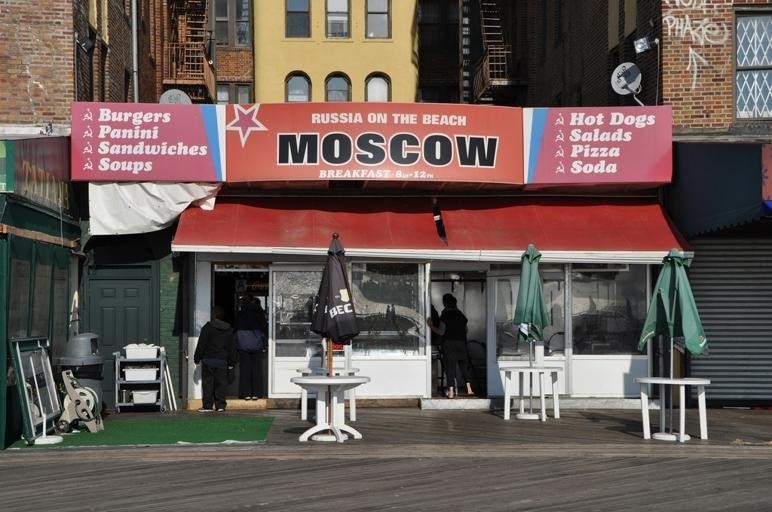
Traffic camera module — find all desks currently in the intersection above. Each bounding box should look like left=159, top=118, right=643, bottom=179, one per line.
left=291, top=376, right=371, bottom=443
left=500, top=366, right=564, bottom=420
left=634, top=376, right=710, bottom=443
left=296, top=367, right=360, bottom=422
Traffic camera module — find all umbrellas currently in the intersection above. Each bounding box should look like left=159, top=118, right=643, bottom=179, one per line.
left=638, top=248, right=709, bottom=435
left=512, top=244, right=551, bottom=416
left=310, top=233, right=362, bottom=435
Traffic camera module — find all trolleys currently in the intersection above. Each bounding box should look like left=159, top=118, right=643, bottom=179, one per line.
left=111, top=348, right=168, bottom=413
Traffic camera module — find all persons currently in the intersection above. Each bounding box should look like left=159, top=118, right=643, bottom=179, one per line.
left=427, top=293, right=475, bottom=399
left=238, top=296, right=267, bottom=400
left=193, top=304, right=239, bottom=413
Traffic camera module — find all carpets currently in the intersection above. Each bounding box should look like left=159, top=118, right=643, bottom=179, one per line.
left=8, top=416, right=275, bottom=448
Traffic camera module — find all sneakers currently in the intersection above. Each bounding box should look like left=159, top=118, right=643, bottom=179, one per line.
left=198, top=408, right=214, bottom=413
left=216, top=408, right=225, bottom=412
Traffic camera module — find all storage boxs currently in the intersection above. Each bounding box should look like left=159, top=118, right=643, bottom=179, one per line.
left=122, top=368, right=160, bottom=381
left=131, top=390, right=159, bottom=403
left=123, top=346, right=160, bottom=359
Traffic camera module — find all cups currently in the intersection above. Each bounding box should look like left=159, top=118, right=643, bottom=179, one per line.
left=535, top=341, right=544, bottom=368
